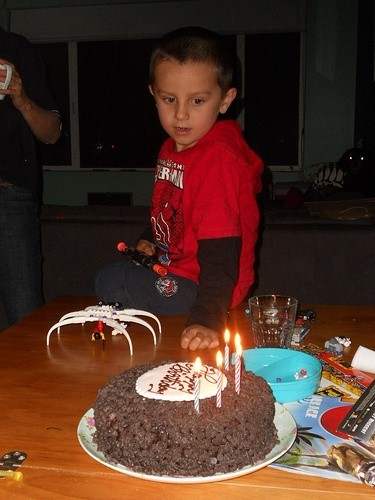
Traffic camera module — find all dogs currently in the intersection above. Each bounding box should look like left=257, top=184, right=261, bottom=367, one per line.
left=301, top=145, right=375, bottom=204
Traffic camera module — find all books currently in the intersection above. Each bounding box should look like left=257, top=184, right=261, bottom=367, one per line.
left=267, top=394, right=375, bottom=483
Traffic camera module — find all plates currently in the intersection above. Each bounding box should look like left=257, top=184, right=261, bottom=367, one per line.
left=77, top=407, right=297, bottom=483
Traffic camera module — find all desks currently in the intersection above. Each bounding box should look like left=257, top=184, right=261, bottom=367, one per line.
left=0, top=295, right=375, bottom=500
left=39, top=205, right=375, bottom=305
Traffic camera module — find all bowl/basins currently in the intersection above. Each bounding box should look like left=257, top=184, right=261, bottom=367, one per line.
left=224, top=348, right=323, bottom=403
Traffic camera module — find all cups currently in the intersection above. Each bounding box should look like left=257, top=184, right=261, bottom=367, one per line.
left=248, top=296, right=298, bottom=350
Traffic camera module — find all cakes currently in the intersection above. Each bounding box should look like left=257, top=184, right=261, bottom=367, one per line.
left=93, top=358, right=276, bottom=474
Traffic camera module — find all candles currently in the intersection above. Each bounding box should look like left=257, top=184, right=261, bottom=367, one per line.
left=193, top=356, right=202, bottom=412
left=216, top=350, right=224, bottom=407
left=234, top=346, right=244, bottom=394
left=224, top=329, right=231, bottom=370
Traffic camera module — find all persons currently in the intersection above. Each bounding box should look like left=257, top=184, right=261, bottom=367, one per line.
left=0, top=26, right=64, bottom=329
left=95, top=24, right=265, bottom=350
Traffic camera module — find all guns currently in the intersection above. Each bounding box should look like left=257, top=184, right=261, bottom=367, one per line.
left=117, top=242, right=167, bottom=276
